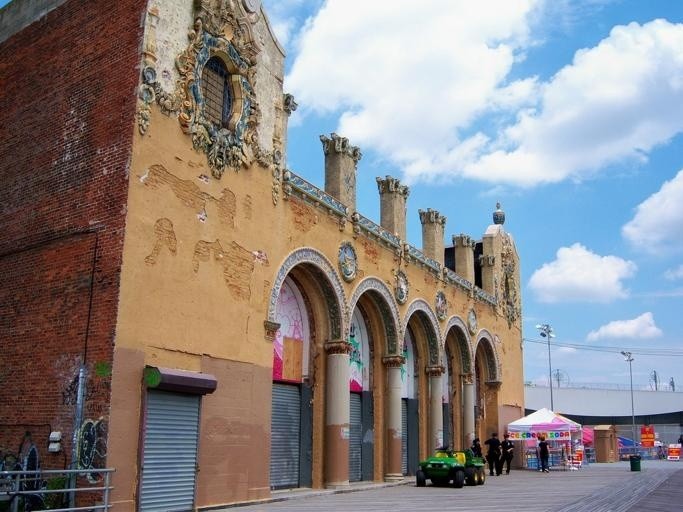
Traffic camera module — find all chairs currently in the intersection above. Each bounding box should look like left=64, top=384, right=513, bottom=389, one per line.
left=434, top=452, right=466, bottom=464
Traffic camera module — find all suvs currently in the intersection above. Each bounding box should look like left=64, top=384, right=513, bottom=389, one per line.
left=416, top=448, right=487, bottom=488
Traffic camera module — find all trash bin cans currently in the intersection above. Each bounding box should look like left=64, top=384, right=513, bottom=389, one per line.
left=630, top=455, right=640, bottom=471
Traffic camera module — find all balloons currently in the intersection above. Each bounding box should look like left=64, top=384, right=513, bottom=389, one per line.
left=554, top=432, right=559, bottom=438
left=541, top=432, right=548, bottom=438
left=515, top=432, right=520, bottom=438
left=549, top=432, right=554, bottom=438
left=564, top=432, right=569, bottom=437
left=521, top=432, right=526, bottom=438
left=525, top=432, right=531, bottom=438
left=509, top=432, right=515, bottom=438
left=559, top=432, right=564, bottom=437
left=536, top=432, right=540, bottom=438
left=531, top=432, right=536, bottom=438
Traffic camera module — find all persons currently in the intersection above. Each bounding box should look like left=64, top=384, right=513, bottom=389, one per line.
left=498, top=433, right=515, bottom=476
left=656, top=445, right=664, bottom=456
left=341, top=247, right=354, bottom=275
left=483, top=431, right=500, bottom=476
left=535, top=436, right=542, bottom=472
left=471, top=436, right=486, bottom=465
left=678, top=434, right=682, bottom=448
left=537, top=435, right=550, bottom=473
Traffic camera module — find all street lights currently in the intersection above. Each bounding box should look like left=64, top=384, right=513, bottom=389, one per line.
left=535, top=320, right=555, bottom=412
left=622, top=351, right=637, bottom=457
